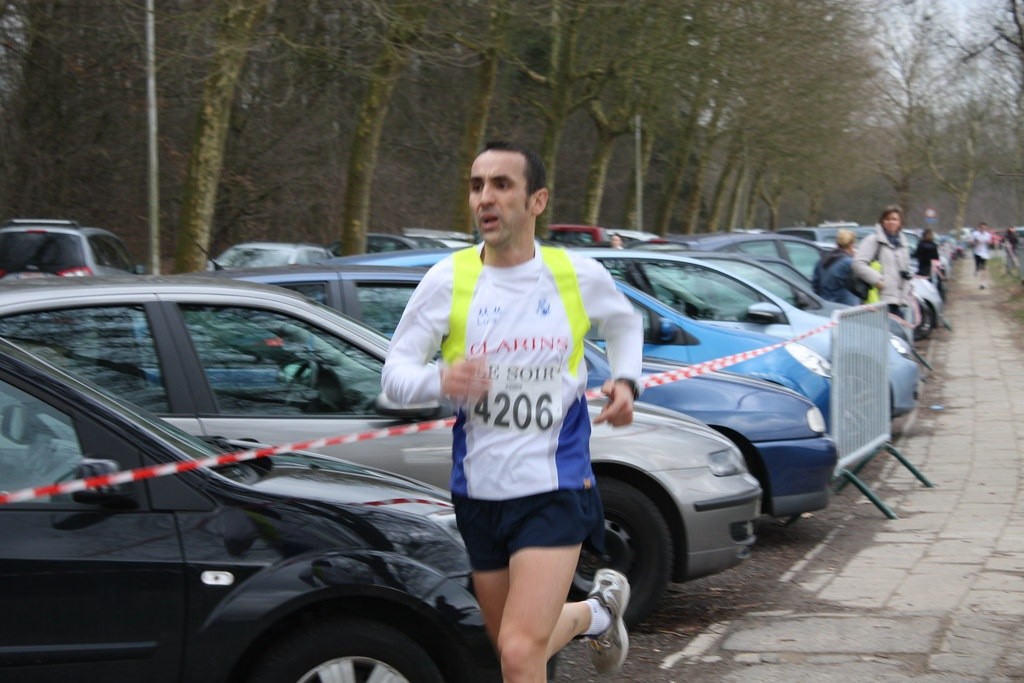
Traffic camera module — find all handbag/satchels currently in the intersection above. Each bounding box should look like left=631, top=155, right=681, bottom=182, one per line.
left=863, top=244, right=882, bottom=304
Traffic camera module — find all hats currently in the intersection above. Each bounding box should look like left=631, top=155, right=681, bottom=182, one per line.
left=837, top=229, right=855, bottom=246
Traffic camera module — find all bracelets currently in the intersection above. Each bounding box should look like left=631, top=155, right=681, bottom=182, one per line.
left=617, top=378, right=639, bottom=400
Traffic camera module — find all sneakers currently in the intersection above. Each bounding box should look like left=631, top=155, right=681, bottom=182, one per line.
left=572, top=568, right=631, bottom=677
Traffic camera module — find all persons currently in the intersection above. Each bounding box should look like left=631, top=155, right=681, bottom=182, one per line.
left=912, top=228, right=944, bottom=293
left=380, top=140, right=643, bottom=683
left=968, top=222, right=994, bottom=289
left=1006, top=226, right=1019, bottom=250
left=852, top=204, right=912, bottom=306
left=611, top=234, right=624, bottom=250
left=815, top=230, right=862, bottom=307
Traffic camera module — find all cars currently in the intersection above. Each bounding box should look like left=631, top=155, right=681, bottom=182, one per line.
left=209, top=223, right=1024, bottom=423
left=136, top=267, right=839, bottom=522
left=0, top=331, right=557, bottom=682
left=201, top=241, right=327, bottom=274
left=0, top=268, right=765, bottom=636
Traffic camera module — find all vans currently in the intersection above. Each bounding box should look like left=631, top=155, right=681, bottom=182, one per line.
left=0, top=212, right=139, bottom=282
